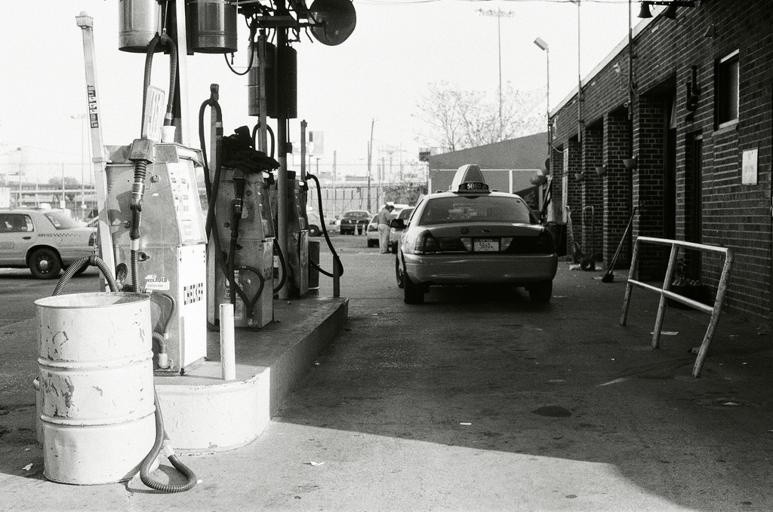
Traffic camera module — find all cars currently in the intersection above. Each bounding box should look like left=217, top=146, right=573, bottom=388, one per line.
left=306, top=205, right=337, bottom=237
left=391, top=164, right=558, bottom=303
left=340, top=204, right=416, bottom=254
left=0, top=208, right=97, bottom=279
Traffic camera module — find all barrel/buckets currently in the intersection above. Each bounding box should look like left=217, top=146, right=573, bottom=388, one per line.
left=33, top=291, right=161, bottom=484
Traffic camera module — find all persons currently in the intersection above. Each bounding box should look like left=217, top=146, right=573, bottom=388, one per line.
left=377, top=204, right=394, bottom=255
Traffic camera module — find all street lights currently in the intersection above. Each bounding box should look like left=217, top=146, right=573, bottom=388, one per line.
left=477, top=6, right=516, bottom=142
left=533, top=36, right=549, bottom=114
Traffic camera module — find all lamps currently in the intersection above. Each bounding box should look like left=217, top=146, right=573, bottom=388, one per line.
left=635, top=0, right=695, bottom=20
